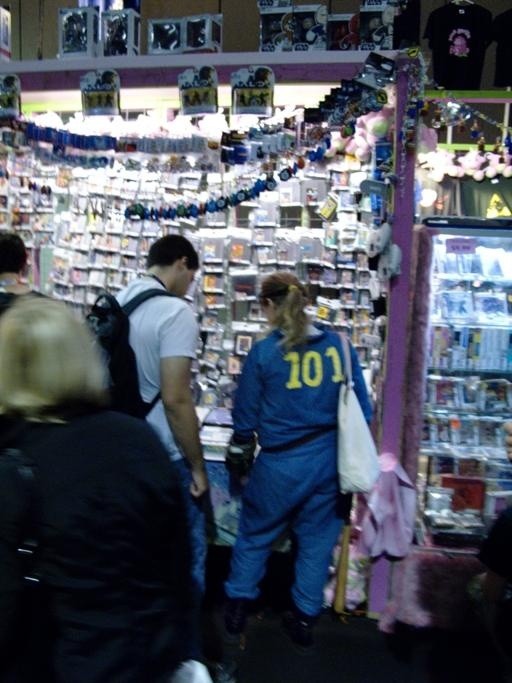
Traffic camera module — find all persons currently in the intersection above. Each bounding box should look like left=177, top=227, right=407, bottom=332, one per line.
left=2, top=297, right=200, bottom=682
left=1, top=232, right=44, bottom=312
left=116, top=235, right=212, bottom=683
left=213, top=272, right=375, bottom=656
left=474, top=502, right=512, bottom=681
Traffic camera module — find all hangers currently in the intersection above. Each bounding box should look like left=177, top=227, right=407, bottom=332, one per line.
left=432, top=0, right=493, bottom=16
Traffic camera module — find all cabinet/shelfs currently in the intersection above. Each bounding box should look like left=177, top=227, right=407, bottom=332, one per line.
left=389, top=215, right=512, bottom=635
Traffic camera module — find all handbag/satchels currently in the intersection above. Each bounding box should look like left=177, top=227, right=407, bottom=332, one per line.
left=337, top=384, right=378, bottom=495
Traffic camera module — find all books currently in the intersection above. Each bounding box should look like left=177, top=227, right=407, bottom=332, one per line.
left=194, top=404, right=258, bottom=454
left=417, top=236, right=512, bottom=547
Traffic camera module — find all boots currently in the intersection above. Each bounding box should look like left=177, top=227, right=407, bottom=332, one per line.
left=280, top=611, right=317, bottom=656
left=221, top=599, right=249, bottom=646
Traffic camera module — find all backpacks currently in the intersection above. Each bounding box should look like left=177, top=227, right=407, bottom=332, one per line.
left=86, top=289, right=172, bottom=420
